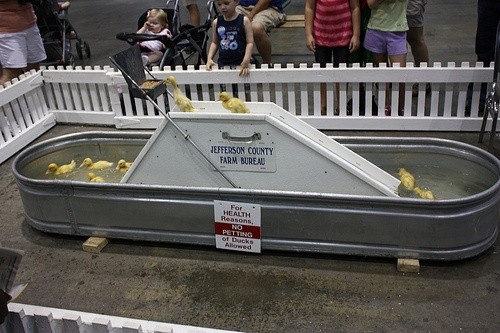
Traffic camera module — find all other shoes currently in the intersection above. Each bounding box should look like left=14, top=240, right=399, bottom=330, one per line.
left=399, top=106, right=405, bottom=116
left=385, top=104, right=391, bottom=115
left=412, top=82, right=432, bottom=97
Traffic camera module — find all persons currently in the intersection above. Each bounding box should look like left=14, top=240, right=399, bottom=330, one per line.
left=206, top=0, right=254, bottom=101
left=464, top=0, right=500, bottom=118
left=167, top=0, right=200, bottom=28
left=136, top=9, right=173, bottom=69
left=235, top=0, right=290, bottom=68
left=0, top=0, right=47, bottom=88
left=305, top=0, right=431, bottom=116
left=51, top=0, right=76, bottom=39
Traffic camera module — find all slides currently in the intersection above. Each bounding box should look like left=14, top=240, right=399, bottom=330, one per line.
left=119, top=100, right=405, bottom=198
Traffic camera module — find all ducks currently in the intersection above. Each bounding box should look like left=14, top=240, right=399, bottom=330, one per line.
left=86, top=171, right=105, bottom=183
left=115, top=158, right=133, bottom=171
left=163, top=75, right=195, bottom=113
left=44, top=159, right=76, bottom=176
left=79, top=156, right=114, bottom=171
left=216, top=90, right=250, bottom=115
left=396, top=166, right=435, bottom=199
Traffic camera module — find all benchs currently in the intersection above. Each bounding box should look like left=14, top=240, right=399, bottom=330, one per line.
left=251, top=14, right=315, bottom=57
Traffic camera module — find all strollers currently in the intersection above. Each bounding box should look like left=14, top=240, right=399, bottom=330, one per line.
left=116, top=0, right=218, bottom=72
left=30, top=0, right=92, bottom=67
